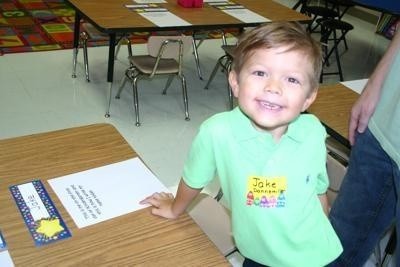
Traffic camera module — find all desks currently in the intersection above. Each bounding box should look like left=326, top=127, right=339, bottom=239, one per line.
left=0, top=122, right=235, bottom=266
left=305, top=77, right=373, bottom=148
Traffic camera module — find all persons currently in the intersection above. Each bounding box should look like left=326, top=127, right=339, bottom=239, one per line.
left=328, top=22, right=400, bottom=267
left=138, top=17, right=346, bottom=266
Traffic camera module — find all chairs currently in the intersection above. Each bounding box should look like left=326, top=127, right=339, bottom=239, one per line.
left=68, top=0, right=355, bottom=127
left=182, top=193, right=239, bottom=261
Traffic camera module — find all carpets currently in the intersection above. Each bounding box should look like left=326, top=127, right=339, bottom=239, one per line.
left=0, top=0, right=246, bottom=55
